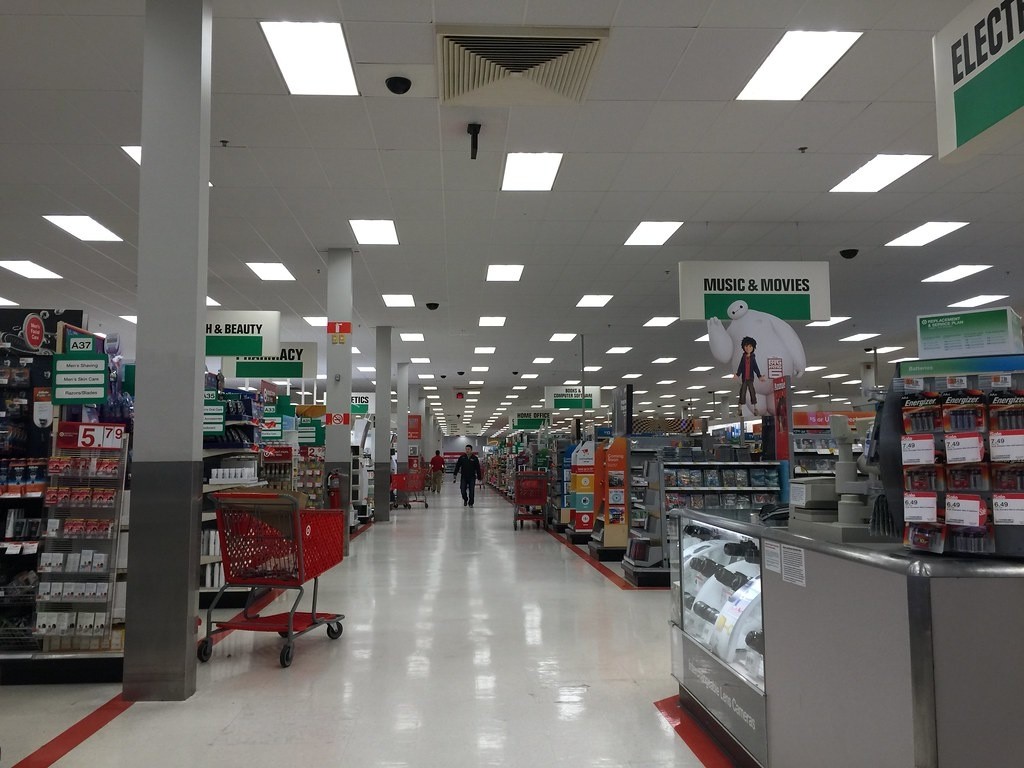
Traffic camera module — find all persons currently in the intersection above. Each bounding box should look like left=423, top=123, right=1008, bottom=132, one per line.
left=452, top=445, right=482, bottom=508
left=429, top=449, right=445, bottom=494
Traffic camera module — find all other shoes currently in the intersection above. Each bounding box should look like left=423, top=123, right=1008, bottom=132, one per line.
left=468, top=502, right=473, bottom=508
left=464, top=500, right=467, bottom=506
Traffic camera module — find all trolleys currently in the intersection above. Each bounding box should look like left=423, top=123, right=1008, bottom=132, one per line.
left=390, top=469, right=429, bottom=510
left=513, top=471, right=548, bottom=531
left=197, top=493, right=346, bottom=667
left=475, top=464, right=486, bottom=489
left=424, top=467, right=433, bottom=490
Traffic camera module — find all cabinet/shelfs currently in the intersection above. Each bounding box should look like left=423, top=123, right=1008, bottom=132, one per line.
left=0, top=307, right=402, bottom=686
left=476, top=389, right=863, bottom=587
